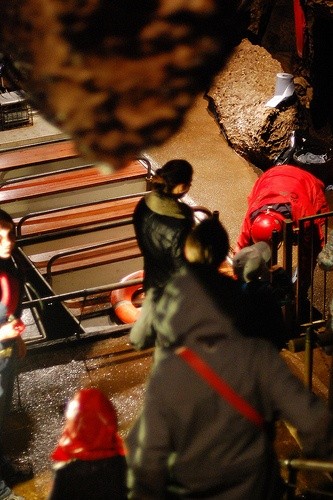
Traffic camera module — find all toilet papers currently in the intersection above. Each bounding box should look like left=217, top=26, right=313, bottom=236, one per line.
left=263, top=72, right=296, bottom=108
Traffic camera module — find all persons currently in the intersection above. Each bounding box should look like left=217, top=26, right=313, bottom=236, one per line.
left=229, top=164, right=331, bottom=326
left=237, top=256, right=285, bottom=340
left=131, top=160, right=195, bottom=304
left=0, top=208, right=22, bottom=329
left=180, top=216, right=246, bottom=329
left=126, top=275, right=333, bottom=499
left=47, top=389, right=128, bottom=500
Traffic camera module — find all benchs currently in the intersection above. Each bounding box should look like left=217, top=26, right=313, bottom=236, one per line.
left=0, top=137, right=239, bottom=331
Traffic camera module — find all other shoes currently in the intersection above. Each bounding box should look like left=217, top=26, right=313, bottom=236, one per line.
left=2, top=458, right=34, bottom=485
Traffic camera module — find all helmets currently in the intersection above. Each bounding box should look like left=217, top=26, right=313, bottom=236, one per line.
left=251, top=210, right=287, bottom=250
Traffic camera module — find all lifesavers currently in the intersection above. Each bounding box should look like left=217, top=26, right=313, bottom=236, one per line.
left=111, top=270, right=146, bottom=324
